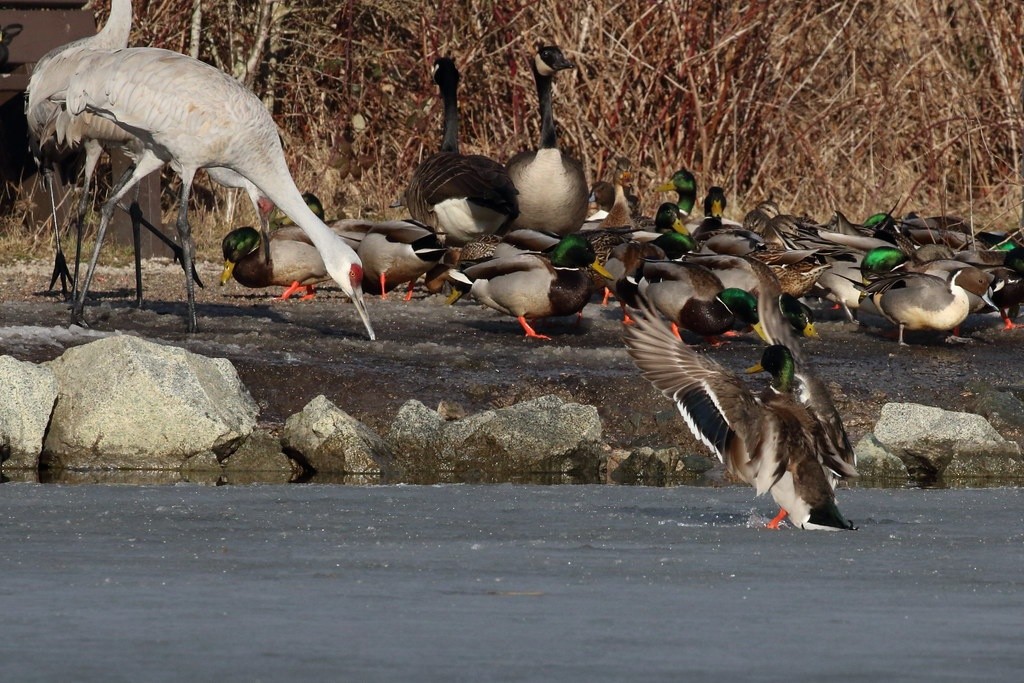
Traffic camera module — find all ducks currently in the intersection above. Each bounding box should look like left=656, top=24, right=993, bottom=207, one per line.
left=221, top=168, right=1024, bottom=530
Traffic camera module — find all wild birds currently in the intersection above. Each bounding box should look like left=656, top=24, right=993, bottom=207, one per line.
left=28, top=0, right=375, bottom=345
left=403, top=45, right=589, bottom=268
